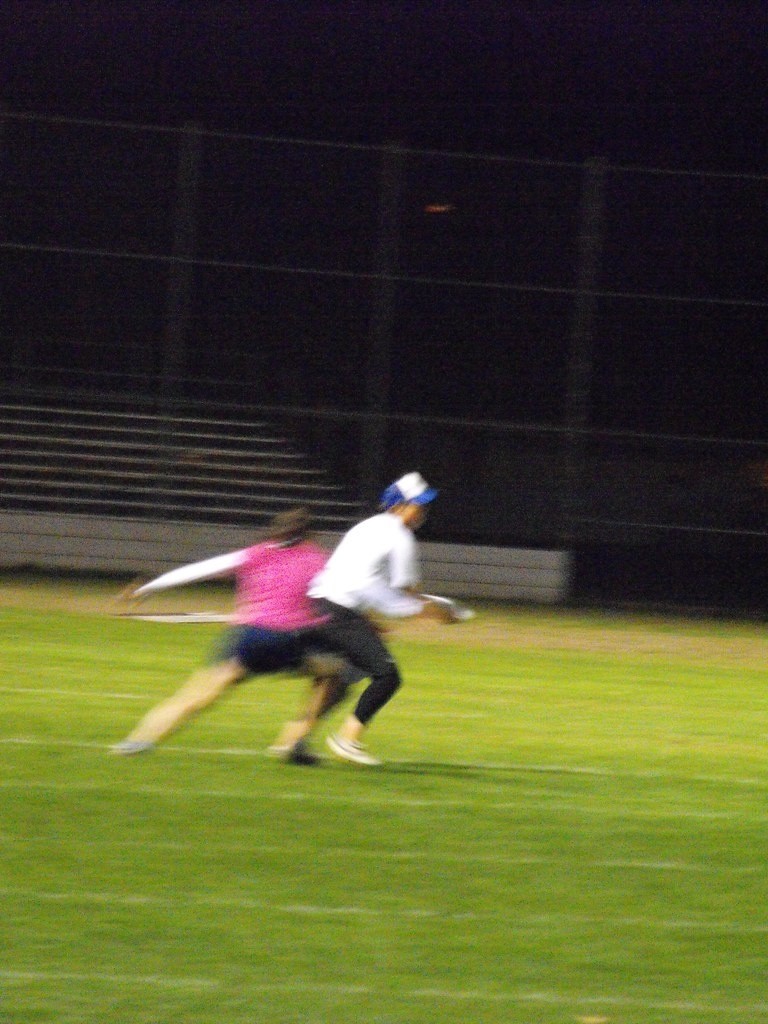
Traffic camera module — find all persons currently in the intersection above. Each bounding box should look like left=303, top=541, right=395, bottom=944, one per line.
left=113, top=472, right=465, bottom=765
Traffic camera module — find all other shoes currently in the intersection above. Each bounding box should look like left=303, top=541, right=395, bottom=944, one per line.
left=330, top=737, right=379, bottom=765
left=285, top=751, right=319, bottom=764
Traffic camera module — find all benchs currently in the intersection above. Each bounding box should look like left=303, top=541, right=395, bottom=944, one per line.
left=0, top=401, right=369, bottom=533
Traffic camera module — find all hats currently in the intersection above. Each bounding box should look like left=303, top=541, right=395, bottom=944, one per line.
left=385, top=472, right=437, bottom=503
